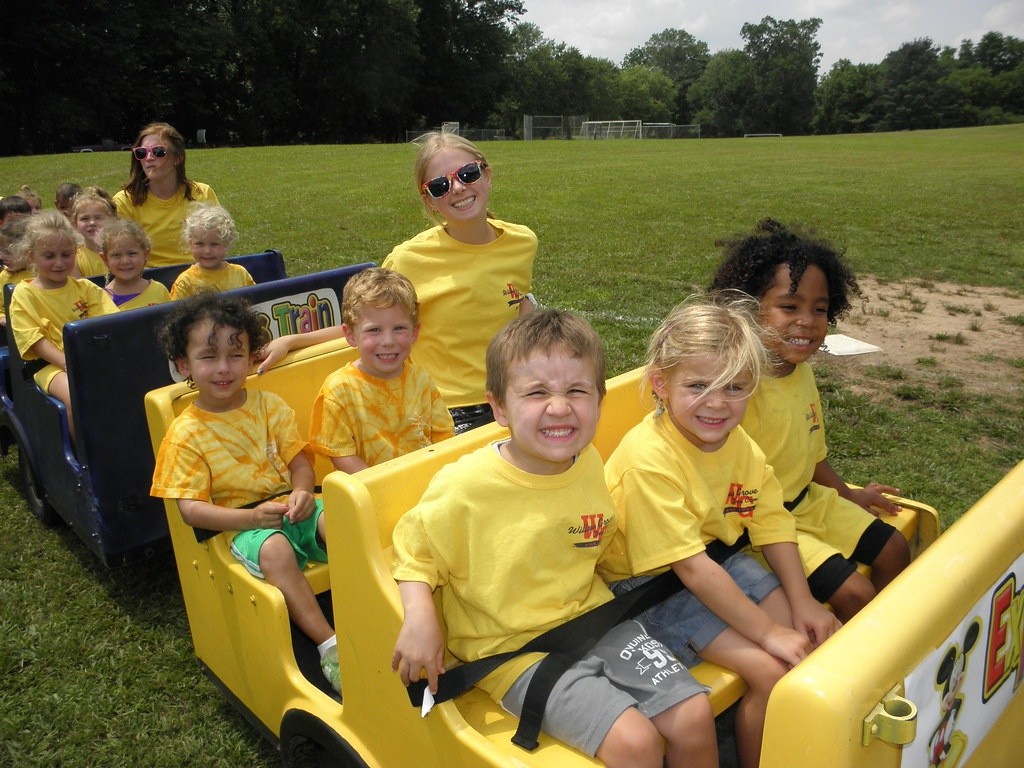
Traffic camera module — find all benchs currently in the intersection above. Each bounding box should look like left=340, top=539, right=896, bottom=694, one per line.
left=63, top=263, right=379, bottom=558
left=3, top=247, right=285, bottom=457
left=322, top=366, right=941, bottom=768
left=145, top=336, right=359, bottom=738
left=759, top=458, right=1024, bottom=768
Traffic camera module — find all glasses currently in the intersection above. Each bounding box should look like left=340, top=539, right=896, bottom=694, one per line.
left=133, top=145, right=175, bottom=159
left=421, top=159, right=487, bottom=199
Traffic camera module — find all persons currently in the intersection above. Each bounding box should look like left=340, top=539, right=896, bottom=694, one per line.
left=1, top=122, right=259, bottom=445
left=143, top=124, right=540, bottom=704
left=392, top=218, right=909, bottom=765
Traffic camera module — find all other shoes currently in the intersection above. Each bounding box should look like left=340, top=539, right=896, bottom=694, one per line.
left=321, top=646, right=344, bottom=698
